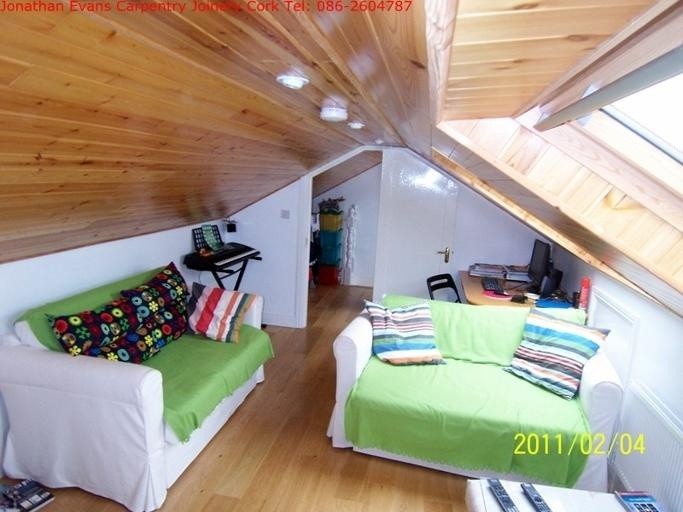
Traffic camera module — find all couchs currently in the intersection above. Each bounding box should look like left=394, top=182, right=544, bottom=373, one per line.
left=326, top=292, right=624, bottom=493
left=0, top=260, right=274, bottom=511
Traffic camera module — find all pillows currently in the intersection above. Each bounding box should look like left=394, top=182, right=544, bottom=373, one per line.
left=44, top=298, right=161, bottom=364
left=185, top=282, right=256, bottom=344
left=120, top=261, right=190, bottom=349
left=504, top=305, right=611, bottom=402
left=362, top=298, right=448, bottom=365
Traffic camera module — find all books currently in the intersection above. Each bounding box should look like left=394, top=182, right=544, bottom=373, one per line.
left=2, top=478, right=56, bottom=512
left=468, top=263, right=531, bottom=281
left=614, top=490, right=670, bottom=511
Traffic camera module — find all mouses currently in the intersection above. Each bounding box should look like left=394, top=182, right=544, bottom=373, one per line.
left=495, top=289, right=511, bottom=295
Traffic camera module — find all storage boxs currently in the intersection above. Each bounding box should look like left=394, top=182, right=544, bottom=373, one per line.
left=319, top=213, right=343, bottom=286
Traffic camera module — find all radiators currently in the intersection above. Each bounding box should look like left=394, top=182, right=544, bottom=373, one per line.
left=609, top=373, right=682, bottom=512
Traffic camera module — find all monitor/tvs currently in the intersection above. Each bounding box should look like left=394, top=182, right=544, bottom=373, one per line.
left=527, top=239, right=550, bottom=295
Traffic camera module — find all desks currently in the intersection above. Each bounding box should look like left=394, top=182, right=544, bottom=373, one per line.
left=465, top=479, right=665, bottom=510
left=459, top=270, right=578, bottom=309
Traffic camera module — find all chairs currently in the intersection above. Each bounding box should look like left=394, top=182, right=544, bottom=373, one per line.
left=427, top=273, right=461, bottom=303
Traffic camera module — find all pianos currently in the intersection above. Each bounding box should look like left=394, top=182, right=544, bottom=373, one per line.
left=183, top=242, right=260, bottom=271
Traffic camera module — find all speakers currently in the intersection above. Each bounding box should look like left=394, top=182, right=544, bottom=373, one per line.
left=550, top=268, right=563, bottom=290
left=539, top=276, right=553, bottom=299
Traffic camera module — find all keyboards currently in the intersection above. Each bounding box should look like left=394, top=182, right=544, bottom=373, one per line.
left=482, top=277, right=501, bottom=291
